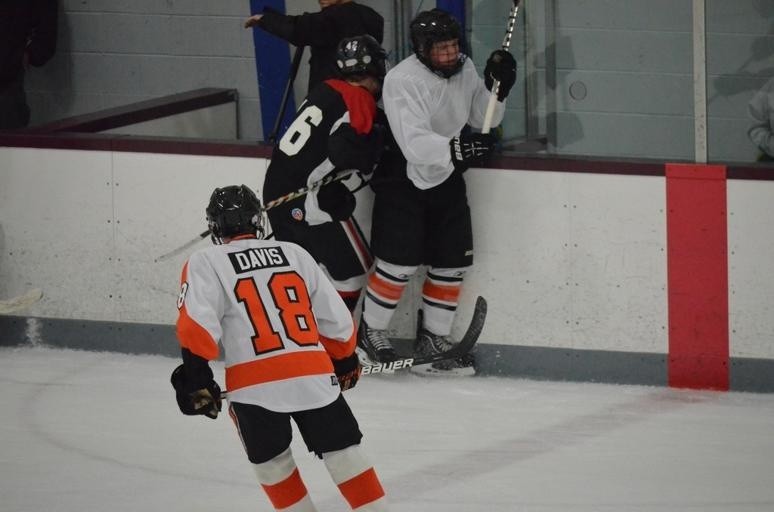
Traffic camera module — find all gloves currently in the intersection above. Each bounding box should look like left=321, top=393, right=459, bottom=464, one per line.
left=331, top=352, right=360, bottom=392
left=484, top=50, right=516, bottom=102
left=171, top=364, right=221, bottom=419
left=450, top=133, right=498, bottom=172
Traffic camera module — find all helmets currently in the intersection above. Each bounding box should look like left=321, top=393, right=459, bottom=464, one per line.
left=336, top=33, right=386, bottom=77
left=207, top=185, right=261, bottom=236
left=410, top=8, right=466, bottom=78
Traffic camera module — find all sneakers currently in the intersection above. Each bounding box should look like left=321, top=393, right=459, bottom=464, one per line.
left=412, top=331, right=474, bottom=367
left=356, top=314, right=399, bottom=363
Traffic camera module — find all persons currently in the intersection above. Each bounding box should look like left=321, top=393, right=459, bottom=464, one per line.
left=244, top=0, right=384, bottom=96
left=352, top=7, right=516, bottom=368
left=171, top=184, right=389, bottom=512
left=263, top=33, right=374, bottom=337
left=746, top=75, right=774, bottom=166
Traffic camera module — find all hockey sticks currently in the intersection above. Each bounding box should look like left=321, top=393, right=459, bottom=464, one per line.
left=0, top=290, right=45, bottom=316
left=219, top=296, right=487, bottom=404
left=155, top=171, right=358, bottom=262
